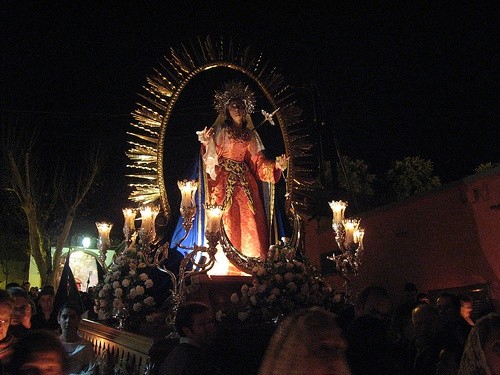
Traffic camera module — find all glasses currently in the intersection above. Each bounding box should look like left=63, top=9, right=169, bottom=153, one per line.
left=187, top=318, right=215, bottom=328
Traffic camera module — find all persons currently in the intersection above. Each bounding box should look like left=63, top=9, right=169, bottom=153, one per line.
left=331, top=282, right=500, bottom=375
left=258, top=308, right=351, bottom=375
left=0, top=282, right=98, bottom=375
left=198, top=84, right=288, bottom=274
left=157, top=303, right=215, bottom=375
